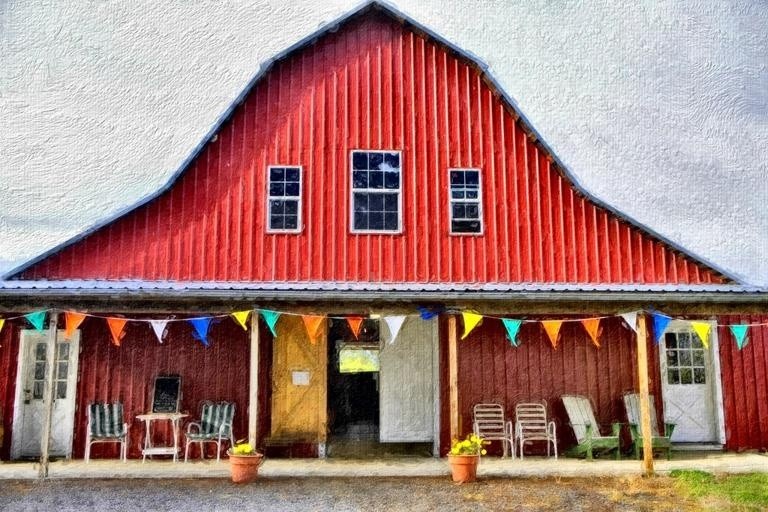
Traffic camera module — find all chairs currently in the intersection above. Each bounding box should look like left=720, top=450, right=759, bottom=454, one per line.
left=562, top=393, right=677, bottom=460
left=184, top=401, right=237, bottom=461
left=84, top=402, right=129, bottom=462
left=471, top=402, right=557, bottom=460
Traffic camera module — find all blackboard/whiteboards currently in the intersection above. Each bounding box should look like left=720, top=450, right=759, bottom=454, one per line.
left=150, top=377, right=181, bottom=416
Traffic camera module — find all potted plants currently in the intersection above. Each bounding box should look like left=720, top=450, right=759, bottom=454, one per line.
left=447, top=431, right=491, bottom=483
left=226, top=444, right=264, bottom=482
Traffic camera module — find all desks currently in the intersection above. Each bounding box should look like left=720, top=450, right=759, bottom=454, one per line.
left=136, top=413, right=188, bottom=462
